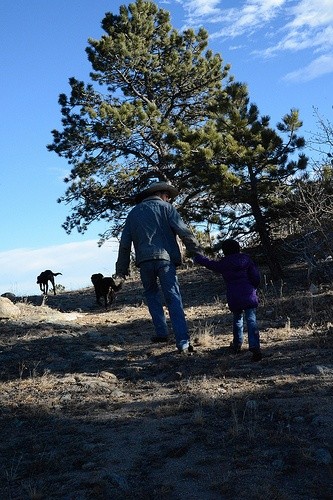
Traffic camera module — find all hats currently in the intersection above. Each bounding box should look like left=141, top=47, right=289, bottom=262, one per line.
left=134, top=182, right=180, bottom=200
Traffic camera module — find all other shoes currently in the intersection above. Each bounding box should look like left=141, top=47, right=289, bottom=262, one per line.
left=177, top=340, right=191, bottom=352
left=219, top=341, right=242, bottom=352
left=150, top=335, right=171, bottom=343
left=250, top=347, right=263, bottom=361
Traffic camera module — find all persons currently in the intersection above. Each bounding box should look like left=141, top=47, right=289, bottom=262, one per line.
left=115, top=182, right=206, bottom=355
left=191, top=239, right=267, bottom=362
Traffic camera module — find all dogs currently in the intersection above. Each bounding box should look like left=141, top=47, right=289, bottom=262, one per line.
left=36, top=269, right=62, bottom=296
left=91, top=273, right=124, bottom=307
